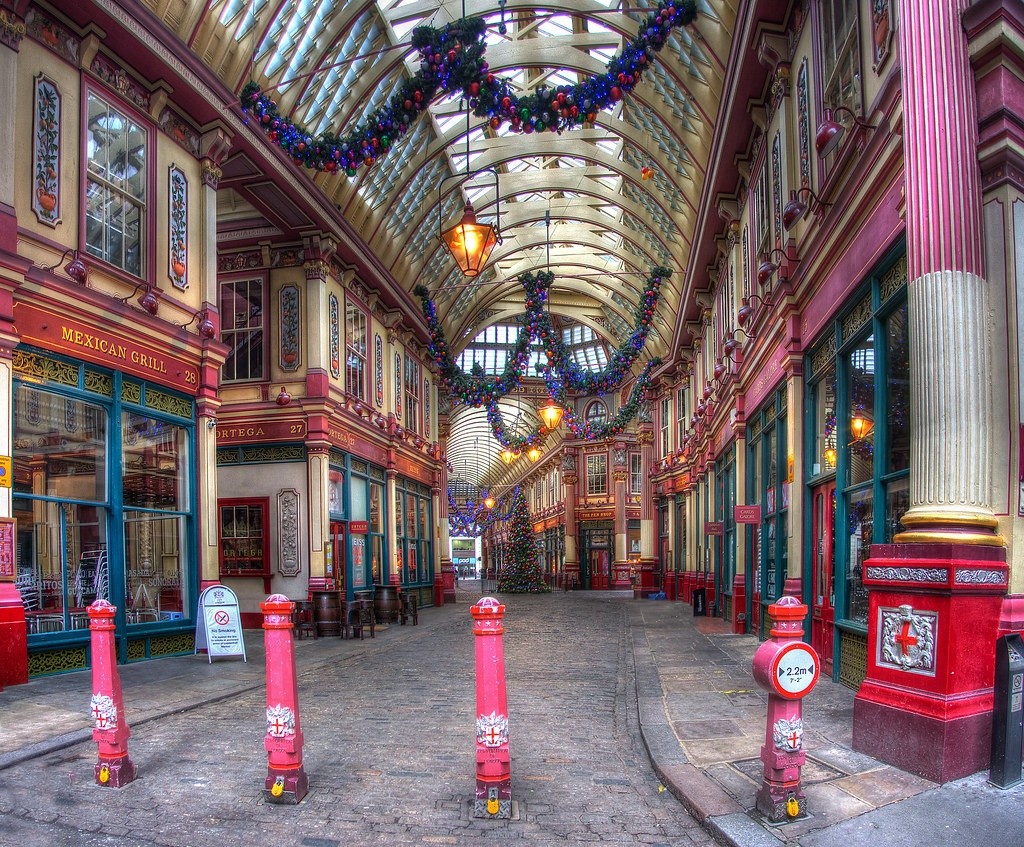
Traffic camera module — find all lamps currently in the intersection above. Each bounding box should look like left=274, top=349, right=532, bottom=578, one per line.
left=438, top=99, right=504, bottom=278
left=757, top=248, right=802, bottom=288
left=382, top=422, right=403, bottom=440
left=738, top=294, right=775, bottom=329
left=421, top=441, right=435, bottom=458
left=435, top=450, right=453, bottom=474
left=498, top=413, right=543, bottom=464
left=538, top=380, right=565, bottom=431
left=175, top=311, right=215, bottom=340
left=403, top=432, right=423, bottom=450
left=483, top=436, right=496, bottom=509
left=815, top=105, right=877, bottom=159
left=484, top=501, right=564, bottom=542
left=276, top=387, right=291, bottom=406
left=114, top=281, right=159, bottom=316
left=338, top=396, right=363, bottom=417
left=361, top=410, right=385, bottom=431
left=782, top=187, right=835, bottom=232
left=43, top=248, right=87, bottom=284
left=580, top=329, right=874, bottom=509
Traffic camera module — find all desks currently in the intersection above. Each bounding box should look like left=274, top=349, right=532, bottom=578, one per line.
left=374, top=584, right=400, bottom=625
left=305, top=589, right=347, bottom=637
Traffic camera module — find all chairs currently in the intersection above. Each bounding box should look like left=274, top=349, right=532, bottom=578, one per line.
left=14, top=542, right=159, bottom=634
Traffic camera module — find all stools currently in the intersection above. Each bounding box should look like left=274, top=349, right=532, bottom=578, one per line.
left=340, top=601, right=364, bottom=641
left=400, top=593, right=418, bottom=627
left=359, top=600, right=375, bottom=638
left=292, top=601, right=318, bottom=641
